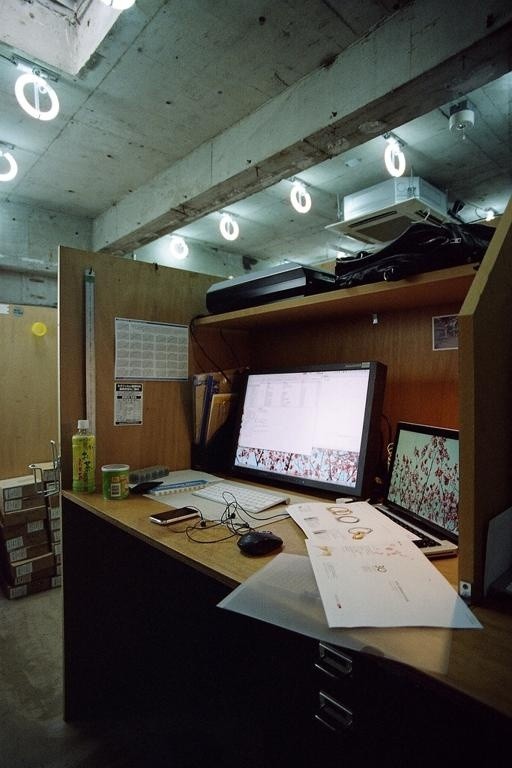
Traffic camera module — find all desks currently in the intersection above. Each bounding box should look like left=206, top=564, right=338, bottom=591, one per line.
left=61, top=196, right=512, bottom=768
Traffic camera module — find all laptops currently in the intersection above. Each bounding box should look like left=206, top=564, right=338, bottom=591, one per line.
left=295, top=420, right=459, bottom=559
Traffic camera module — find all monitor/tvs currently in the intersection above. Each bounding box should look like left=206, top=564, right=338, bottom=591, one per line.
left=226, top=362, right=388, bottom=502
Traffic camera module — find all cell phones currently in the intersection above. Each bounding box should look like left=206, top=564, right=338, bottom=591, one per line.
left=148, top=507, right=200, bottom=524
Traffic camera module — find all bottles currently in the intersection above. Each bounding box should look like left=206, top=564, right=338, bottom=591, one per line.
left=72, top=419, right=96, bottom=493
left=101, top=464, right=130, bottom=502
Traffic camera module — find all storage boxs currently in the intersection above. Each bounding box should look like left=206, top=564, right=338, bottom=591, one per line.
left=0, top=461, right=61, bottom=600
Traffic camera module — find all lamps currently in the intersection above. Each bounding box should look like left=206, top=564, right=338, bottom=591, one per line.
left=0, top=138, right=19, bottom=183
left=168, top=234, right=190, bottom=259
left=219, top=208, right=240, bottom=241
left=384, top=131, right=406, bottom=178
left=288, top=175, right=312, bottom=215
left=448, top=99, right=476, bottom=141
left=15, top=70, right=61, bottom=121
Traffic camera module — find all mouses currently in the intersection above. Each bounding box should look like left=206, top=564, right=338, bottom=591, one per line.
left=236, top=530, right=283, bottom=555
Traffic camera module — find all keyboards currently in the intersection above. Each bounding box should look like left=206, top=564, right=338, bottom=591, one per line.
left=192, top=480, right=290, bottom=516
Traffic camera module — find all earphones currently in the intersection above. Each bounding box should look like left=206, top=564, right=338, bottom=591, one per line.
left=230, top=512, right=235, bottom=519
left=200, top=520, right=207, bottom=528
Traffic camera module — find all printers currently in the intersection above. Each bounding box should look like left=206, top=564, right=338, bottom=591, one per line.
left=204, top=262, right=338, bottom=315
